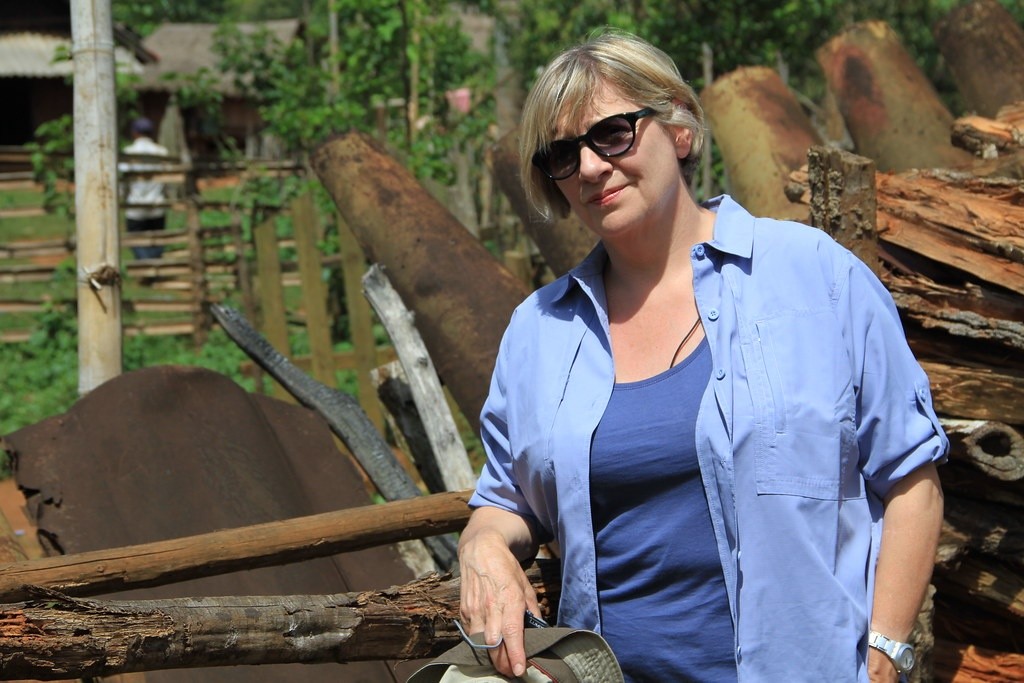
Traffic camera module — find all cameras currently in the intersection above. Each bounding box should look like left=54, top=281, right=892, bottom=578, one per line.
left=524, top=610, right=550, bottom=629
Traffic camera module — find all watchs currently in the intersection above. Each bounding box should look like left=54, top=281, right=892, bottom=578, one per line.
left=869, top=629, right=918, bottom=676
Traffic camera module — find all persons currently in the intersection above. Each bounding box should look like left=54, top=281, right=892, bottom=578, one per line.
left=117, top=117, right=171, bottom=286
left=456, top=30, right=950, bottom=682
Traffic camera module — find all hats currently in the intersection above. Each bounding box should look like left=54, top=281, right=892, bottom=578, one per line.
left=406, top=627, right=625, bottom=683
left=135, top=119, right=153, bottom=134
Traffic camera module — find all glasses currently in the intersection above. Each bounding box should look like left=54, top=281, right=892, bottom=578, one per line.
left=532, top=96, right=673, bottom=180
